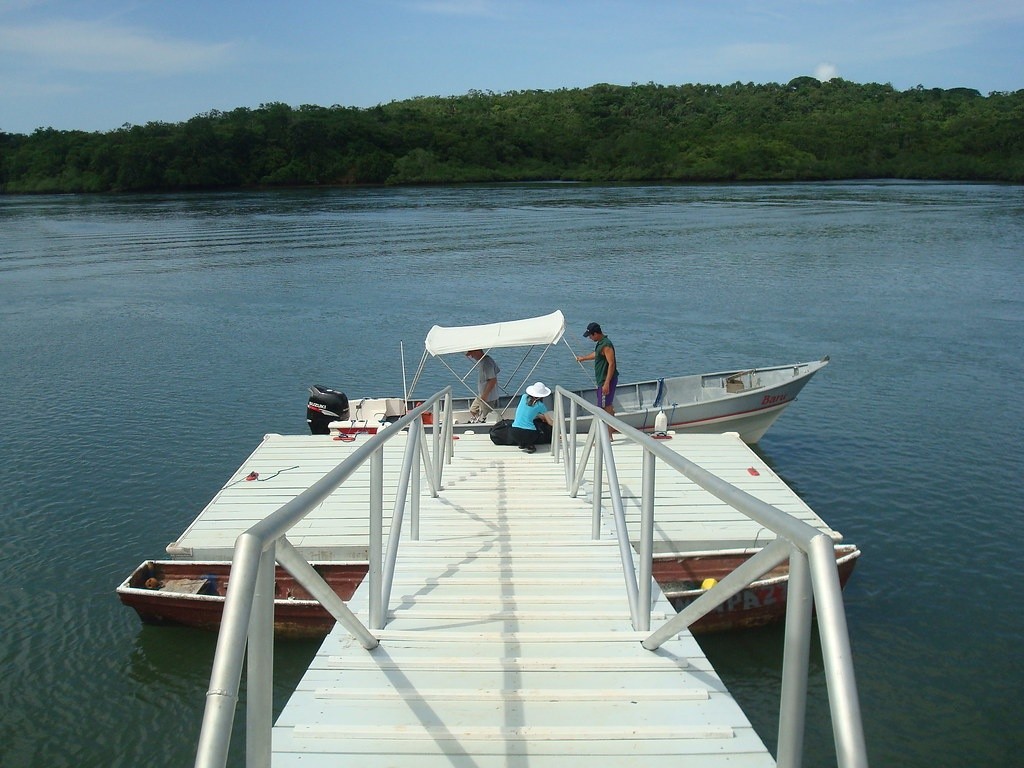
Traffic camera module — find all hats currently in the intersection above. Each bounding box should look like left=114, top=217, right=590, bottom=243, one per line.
left=525, top=381, right=552, bottom=398
left=583, top=322, right=601, bottom=337
left=465, top=352, right=468, bottom=355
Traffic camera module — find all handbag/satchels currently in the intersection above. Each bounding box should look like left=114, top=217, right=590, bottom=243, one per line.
left=489, top=419, right=552, bottom=446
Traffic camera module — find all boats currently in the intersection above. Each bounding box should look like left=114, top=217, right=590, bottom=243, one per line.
left=111, top=543, right=862, bottom=641
left=307, top=310, right=831, bottom=446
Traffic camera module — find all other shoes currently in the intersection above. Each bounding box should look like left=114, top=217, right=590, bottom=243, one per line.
left=518, top=446, right=525, bottom=450
left=526, top=444, right=537, bottom=452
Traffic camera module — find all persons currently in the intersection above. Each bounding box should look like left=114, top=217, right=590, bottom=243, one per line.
left=510, top=382, right=554, bottom=453
left=465, top=349, right=500, bottom=423
left=576, top=322, right=619, bottom=441
